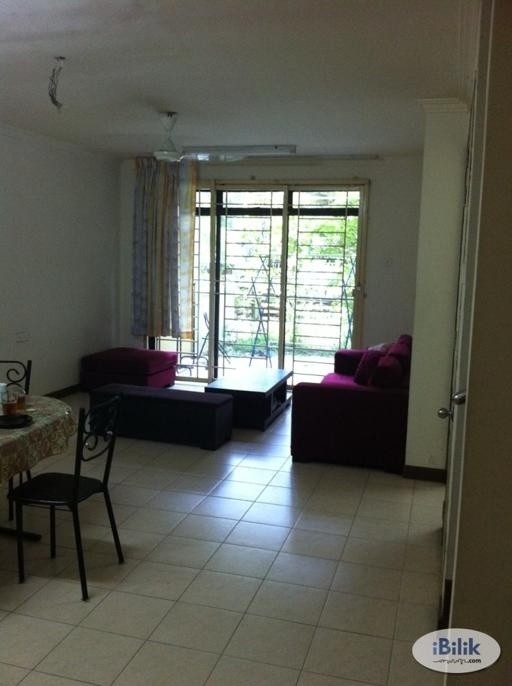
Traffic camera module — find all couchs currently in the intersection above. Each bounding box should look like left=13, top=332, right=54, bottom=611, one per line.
left=291, top=349, right=409, bottom=476
left=80, top=347, right=178, bottom=393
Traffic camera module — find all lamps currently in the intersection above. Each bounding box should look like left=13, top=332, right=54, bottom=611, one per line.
left=153, top=117, right=181, bottom=164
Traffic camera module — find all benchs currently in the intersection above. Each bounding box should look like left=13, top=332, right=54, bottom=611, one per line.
left=90, top=382, right=234, bottom=452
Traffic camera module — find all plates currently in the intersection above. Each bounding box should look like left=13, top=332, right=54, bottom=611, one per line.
left=0, top=414, right=33, bottom=429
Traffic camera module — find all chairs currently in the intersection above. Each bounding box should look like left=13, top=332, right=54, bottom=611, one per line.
left=5, top=396, right=124, bottom=601
left=0, top=359, right=32, bottom=521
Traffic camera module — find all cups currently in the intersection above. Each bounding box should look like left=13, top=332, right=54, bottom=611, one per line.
left=0, top=383, right=25, bottom=415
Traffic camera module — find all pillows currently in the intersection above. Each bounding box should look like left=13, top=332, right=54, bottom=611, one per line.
left=353, top=334, right=411, bottom=388
